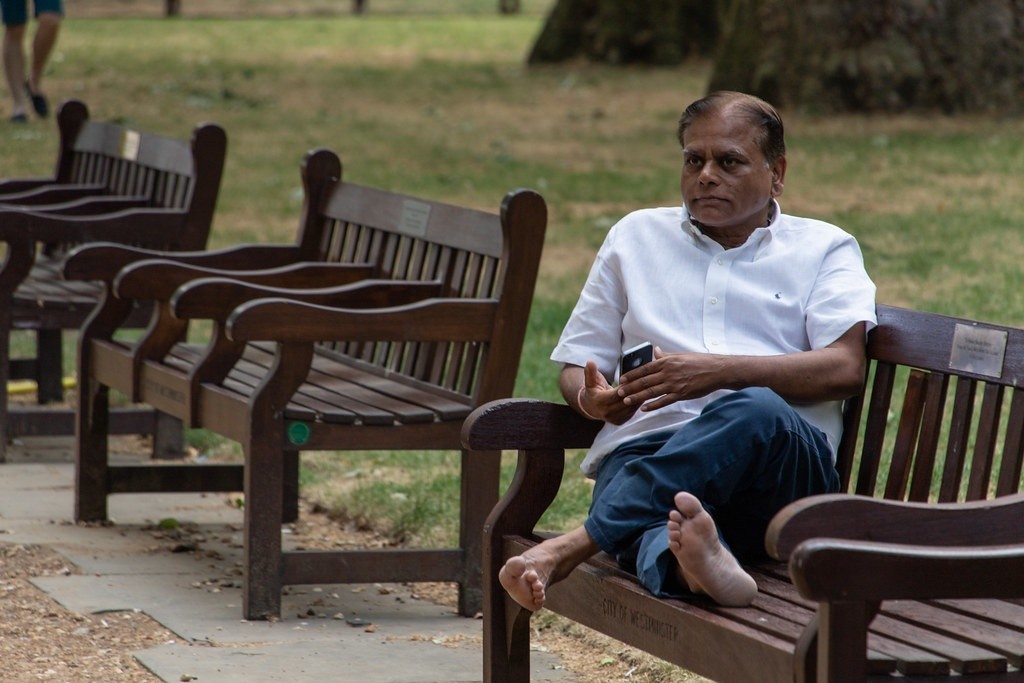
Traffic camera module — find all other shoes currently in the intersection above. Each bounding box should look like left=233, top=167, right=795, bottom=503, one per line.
left=12, top=113, right=26, bottom=121
left=25, top=78, right=47, bottom=117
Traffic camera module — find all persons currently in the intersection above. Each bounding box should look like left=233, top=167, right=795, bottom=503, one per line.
left=498, top=90, right=877, bottom=611
left=0, top=0, right=66, bottom=120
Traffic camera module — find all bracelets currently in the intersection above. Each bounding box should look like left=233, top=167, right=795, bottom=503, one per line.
left=577, top=385, right=600, bottom=420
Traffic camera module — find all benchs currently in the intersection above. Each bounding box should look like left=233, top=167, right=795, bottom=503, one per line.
left=61, top=147, right=548, bottom=622
left=0, top=99, right=228, bottom=463
left=460, top=305, right=1024, bottom=683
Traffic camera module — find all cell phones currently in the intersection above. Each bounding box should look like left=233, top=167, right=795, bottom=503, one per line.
left=620, top=341, right=653, bottom=386
